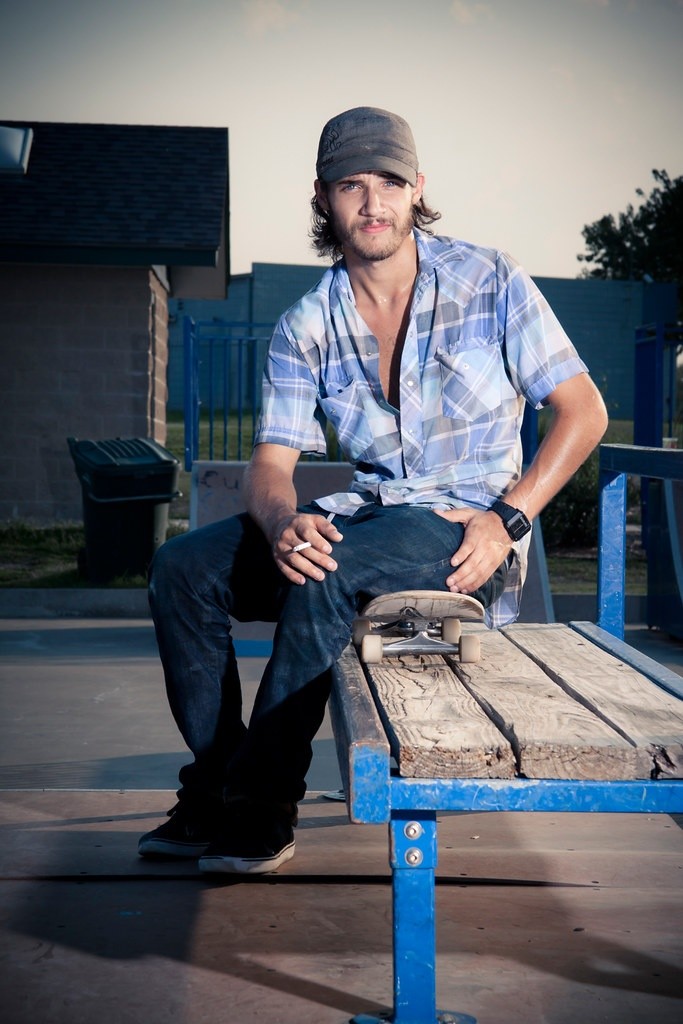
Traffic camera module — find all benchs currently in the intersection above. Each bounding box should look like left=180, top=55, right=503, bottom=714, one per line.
left=328, top=443, right=683, bottom=1024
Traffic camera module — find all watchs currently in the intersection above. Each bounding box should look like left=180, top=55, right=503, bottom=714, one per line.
left=486, top=501, right=533, bottom=541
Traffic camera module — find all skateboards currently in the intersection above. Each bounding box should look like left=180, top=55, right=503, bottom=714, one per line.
left=353, top=587, right=486, bottom=664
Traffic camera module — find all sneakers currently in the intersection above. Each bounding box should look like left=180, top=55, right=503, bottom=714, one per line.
left=138, top=800, right=229, bottom=860
left=198, top=828, right=295, bottom=873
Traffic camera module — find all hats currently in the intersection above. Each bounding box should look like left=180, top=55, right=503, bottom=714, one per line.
left=315, top=105, right=418, bottom=187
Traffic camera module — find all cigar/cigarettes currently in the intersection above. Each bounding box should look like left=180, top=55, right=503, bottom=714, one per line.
left=292, top=540, right=311, bottom=552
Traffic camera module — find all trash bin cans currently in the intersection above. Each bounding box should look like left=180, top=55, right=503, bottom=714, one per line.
left=67, top=434, right=182, bottom=582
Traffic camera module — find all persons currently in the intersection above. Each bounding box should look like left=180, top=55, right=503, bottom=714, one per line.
left=138, top=107, right=610, bottom=869
